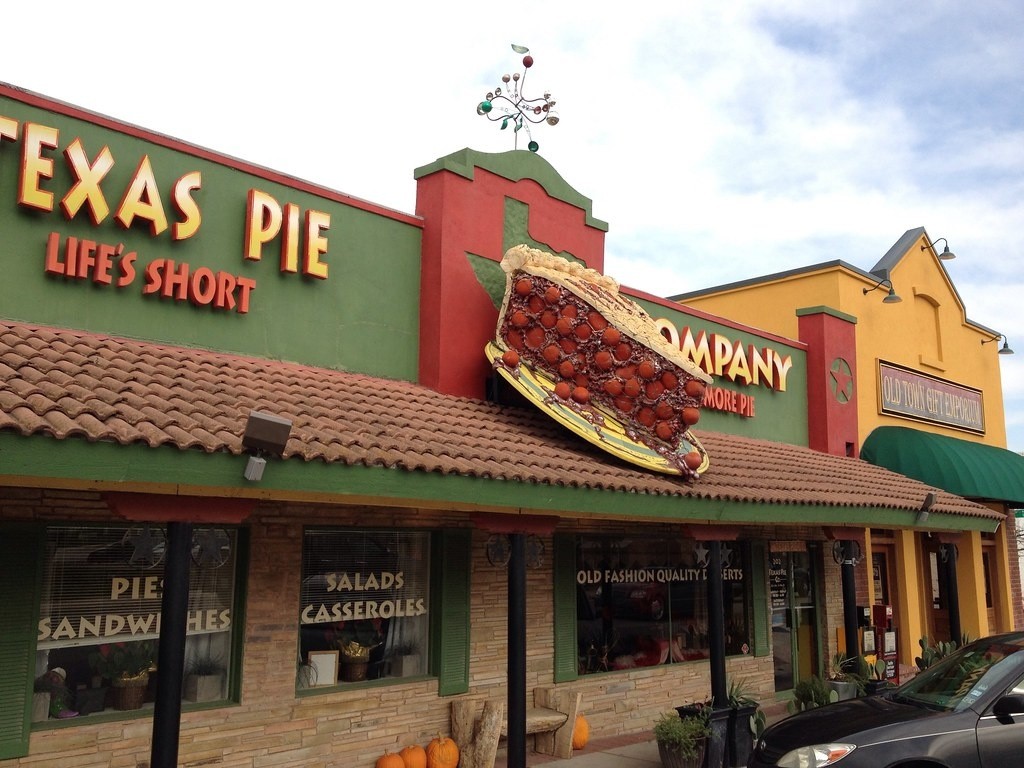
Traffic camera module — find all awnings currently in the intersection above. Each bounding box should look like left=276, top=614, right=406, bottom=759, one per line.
left=857, top=425, right=1024, bottom=508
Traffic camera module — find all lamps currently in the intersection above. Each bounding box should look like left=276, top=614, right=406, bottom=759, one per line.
left=921, top=238, right=956, bottom=259
left=981, top=334, right=1014, bottom=354
left=863, top=279, right=902, bottom=303
left=917, top=492, right=937, bottom=522
left=240, top=410, right=294, bottom=480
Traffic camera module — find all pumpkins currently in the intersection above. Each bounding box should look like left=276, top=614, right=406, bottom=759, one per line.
left=424, top=730, right=459, bottom=768
left=572, top=713, right=589, bottom=750
left=399, top=745, right=427, bottom=768
left=376, top=749, right=405, bottom=768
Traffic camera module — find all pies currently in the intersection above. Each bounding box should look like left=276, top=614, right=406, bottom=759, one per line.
left=495, top=245, right=715, bottom=466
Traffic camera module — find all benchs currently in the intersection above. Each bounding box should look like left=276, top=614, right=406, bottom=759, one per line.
left=451, top=685, right=582, bottom=768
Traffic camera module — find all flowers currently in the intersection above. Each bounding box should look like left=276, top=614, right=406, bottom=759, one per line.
left=34, top=671, right=74, bottom=698
left=87, top=644, right=155, bottom=677
left=685, top=695, right=714, bottom=710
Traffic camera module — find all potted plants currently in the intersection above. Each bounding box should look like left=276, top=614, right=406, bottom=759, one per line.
left=652, top=705, right=714, bottom=768
left=728, top=677, right=766, bottom=768
left=184, top=650, right=227, bottom=703
left=824, top=653, right=869, bottom=702
left=385, top=640, right=420, bottom=677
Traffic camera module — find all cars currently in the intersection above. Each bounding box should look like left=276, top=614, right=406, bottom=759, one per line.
left=594, top=565, right=693, bottom=622
left=747, top=629, right=1024, bottom=768
left=721, top=550, right=810, bottom=596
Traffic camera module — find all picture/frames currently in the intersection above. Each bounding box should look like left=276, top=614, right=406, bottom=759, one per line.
left=308, top=650, right=339, bottom=689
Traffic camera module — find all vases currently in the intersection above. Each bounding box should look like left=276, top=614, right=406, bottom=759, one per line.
left=675, top=705, right=732, bottom=768
left=113, top=677, right=147, bottom=709
left=31, top=693, right=50, bottom=723
left=864, top=679, right=888, bottom=696
